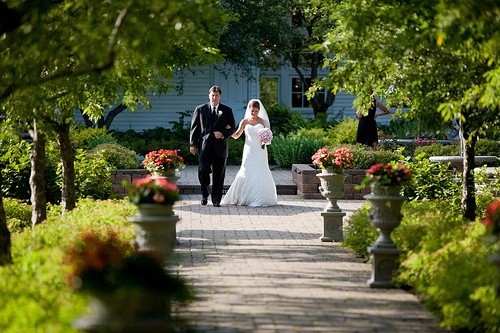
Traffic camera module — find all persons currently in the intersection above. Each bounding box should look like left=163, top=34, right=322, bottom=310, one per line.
left=357, top=86, right=389, bottom=148
left=190, top=85, right=236, bottom=206
left=221, top=99, right=278, bottom=207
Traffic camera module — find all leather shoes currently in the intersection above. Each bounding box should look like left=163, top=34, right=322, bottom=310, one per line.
left=212, top=199, right=220, bottom=207
left=201, top=197, right=207, bottom=205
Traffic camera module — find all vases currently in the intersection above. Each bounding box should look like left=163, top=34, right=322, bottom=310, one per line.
left=153, top=170, right=175, bottom=177
left=139, top=204, right=172, bottom=216
left=321, top=166, right=343, bottom=174
left=369, top=185, right=403, bottom=197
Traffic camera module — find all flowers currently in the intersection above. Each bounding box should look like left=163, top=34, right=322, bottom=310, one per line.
left=142, top=149, right=186, bottom=172
left=311, top=149, right=354, bottom=171
left=367, top=163, right=411, bottom=189
left=123, top=179, right=183, bottom=206
left=258, top=128, right=273, bottom=149
left=217, top=111, right=222, bottom=117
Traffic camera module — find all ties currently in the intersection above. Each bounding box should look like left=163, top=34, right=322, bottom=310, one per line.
left=212, top=106, right=217, bottom=123
